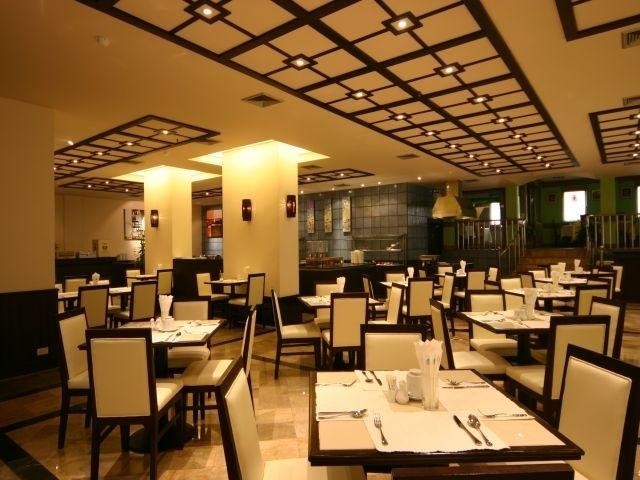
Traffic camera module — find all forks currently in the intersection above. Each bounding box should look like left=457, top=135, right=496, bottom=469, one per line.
left=374, top=415, right=388, bottom=446
left=314, top=377, right=357, bottom=387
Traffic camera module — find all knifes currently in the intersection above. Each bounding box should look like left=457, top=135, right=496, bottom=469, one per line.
left=454, top=414, right=483, bottom=446
left=442, top=385, right=490, bottom=389
left=370, top=370, right=383, bottom=387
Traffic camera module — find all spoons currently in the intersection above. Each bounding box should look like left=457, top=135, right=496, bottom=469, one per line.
left=318, top=407, right=367, bottom=418
left=467, top=414, right=494, bottom=447
left=362, top=371, right=374, bottom=383
left=172, top=332, right=182, bottom=342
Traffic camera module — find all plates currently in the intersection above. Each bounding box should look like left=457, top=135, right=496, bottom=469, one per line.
left=351, top=249, right=365, bottom=264
left=387, top=248, right=402, bottom=253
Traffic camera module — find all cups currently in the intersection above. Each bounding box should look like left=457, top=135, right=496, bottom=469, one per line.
left=219, top=272, right=224, bottom=282
left=421, top=371, right=440, bottom=411
left=91, top=275, right=100, bottom=284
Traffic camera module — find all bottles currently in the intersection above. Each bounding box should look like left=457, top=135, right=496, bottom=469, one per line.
left=492, top=304, right=534, bottom=328
left=406, top=368, right=426, bottom=402
left=306, top=256, right=344, bottom=268
left=395, top=379, right=410, bottom=406
left=456, top=269, right=464, bottom=275
left=314, top=295, right=327, bottom=304
left=539, top=267, right=584, bottom=293
left=149, top=315, right=180, bottom=332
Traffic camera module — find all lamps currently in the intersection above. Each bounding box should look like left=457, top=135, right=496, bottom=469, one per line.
left=151, top=209, right=159, bottom=227
left=286, top=195, right=297, bottom=217
left=242, top=199, right=252, bottom=222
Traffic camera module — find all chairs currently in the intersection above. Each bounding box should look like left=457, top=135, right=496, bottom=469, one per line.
left=59, top=263, right=640, bottom=480
left=468, top=217, right=636, bottom=237
